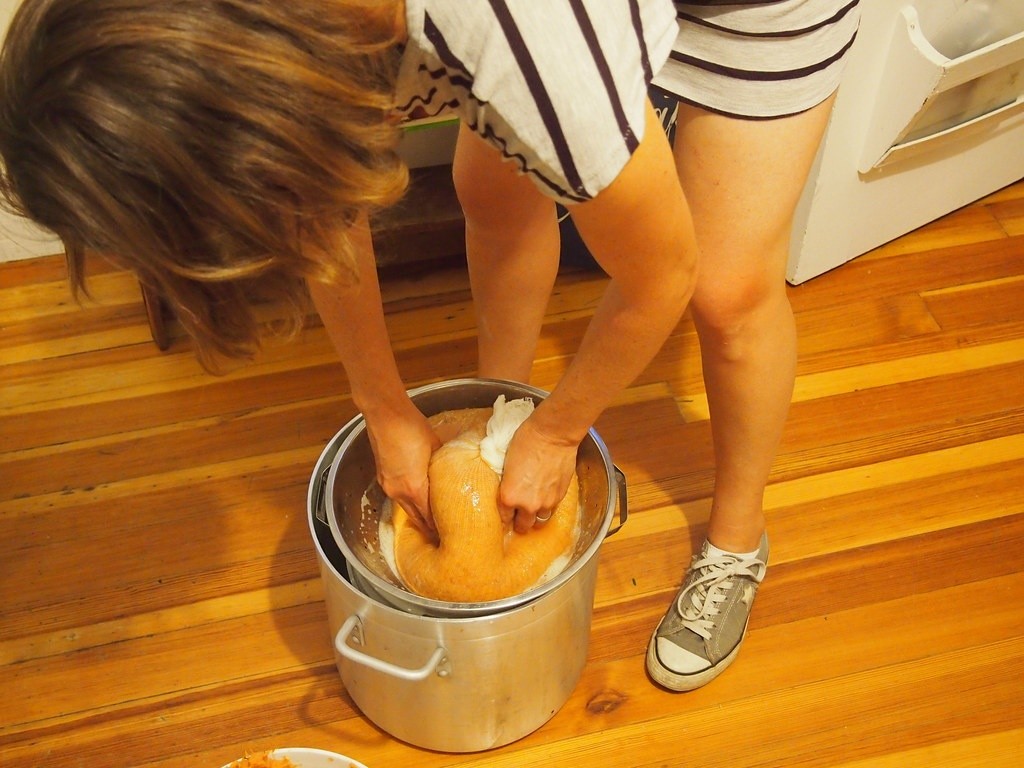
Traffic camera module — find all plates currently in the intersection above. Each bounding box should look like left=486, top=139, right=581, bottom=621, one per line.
left=219, top=744, right=369, bottom=767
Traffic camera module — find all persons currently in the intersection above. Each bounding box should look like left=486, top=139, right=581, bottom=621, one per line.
left=0, top=0, right=866, bottom=694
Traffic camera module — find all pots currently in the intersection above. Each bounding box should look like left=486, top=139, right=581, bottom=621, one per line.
left=314, top=375, right=629, bottom=616
left=308, top=389, right=600, bottom=754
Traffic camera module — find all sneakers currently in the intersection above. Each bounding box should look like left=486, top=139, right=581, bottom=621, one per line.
left=646, top=526, right=770, bottom=691
